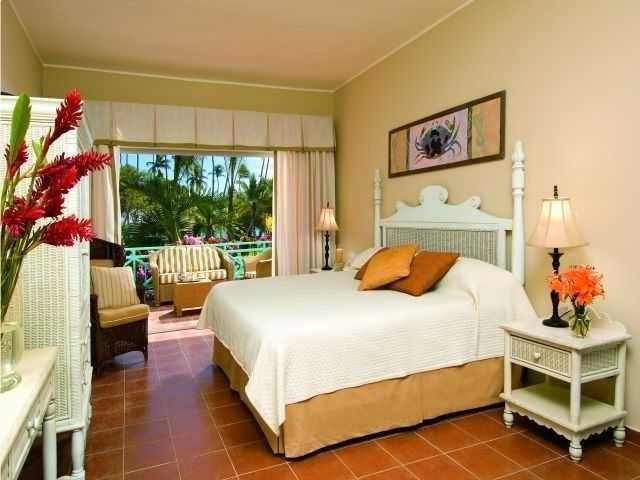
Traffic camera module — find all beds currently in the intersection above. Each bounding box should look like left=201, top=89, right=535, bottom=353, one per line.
left=197, top=139, right=545, bottom=457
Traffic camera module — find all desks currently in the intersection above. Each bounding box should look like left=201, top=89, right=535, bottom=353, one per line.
left=1, top=348, right=60, bottom=480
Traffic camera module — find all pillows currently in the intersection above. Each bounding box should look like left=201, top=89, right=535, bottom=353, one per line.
left=433, top=256, right=521, bottom=301
left=93, top=266, right=140, bottom=309
left=357, top=243, right=421, bottom=291
left=354, top=247, right=388, bottom=280
left=388, top=248, right=459, bottom=296
left=351, top=248, right=384, bottom=269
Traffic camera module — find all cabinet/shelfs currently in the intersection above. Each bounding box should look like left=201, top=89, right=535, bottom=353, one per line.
left=1, top=94, right=95, bottom=480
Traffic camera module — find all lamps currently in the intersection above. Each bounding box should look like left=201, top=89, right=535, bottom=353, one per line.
left=527, top=182, right=589, bottom=328
left=315, top=202, right=340, bottom=271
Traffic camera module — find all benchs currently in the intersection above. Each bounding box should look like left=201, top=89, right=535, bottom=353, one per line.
left=150, top=246, right=233, bottom=306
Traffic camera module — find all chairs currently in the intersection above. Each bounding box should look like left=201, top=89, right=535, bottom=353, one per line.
left=90, top=238, right=149, bottom=374
left=242, top=247, right=272, bottom=279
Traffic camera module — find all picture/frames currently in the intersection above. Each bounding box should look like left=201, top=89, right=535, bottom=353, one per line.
left=388, top=90, right=506, bottom=176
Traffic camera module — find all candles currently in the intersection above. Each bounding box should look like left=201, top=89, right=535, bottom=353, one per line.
left=335, top=248, right=343, bottom=264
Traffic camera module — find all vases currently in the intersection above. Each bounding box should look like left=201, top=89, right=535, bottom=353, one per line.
left=1, top=274, right=24, bottom=393
left=566, top=308, right=591, bottom=338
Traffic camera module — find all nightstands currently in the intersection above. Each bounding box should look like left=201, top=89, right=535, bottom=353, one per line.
left=498, top=319, right=632, bottom=462
left=310, top=266, right=335, bottom=274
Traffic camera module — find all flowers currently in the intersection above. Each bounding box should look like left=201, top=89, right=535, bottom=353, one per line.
left=0, top=86, right=114, bottom=323
left=545, top=264, right=607, bottom=333
